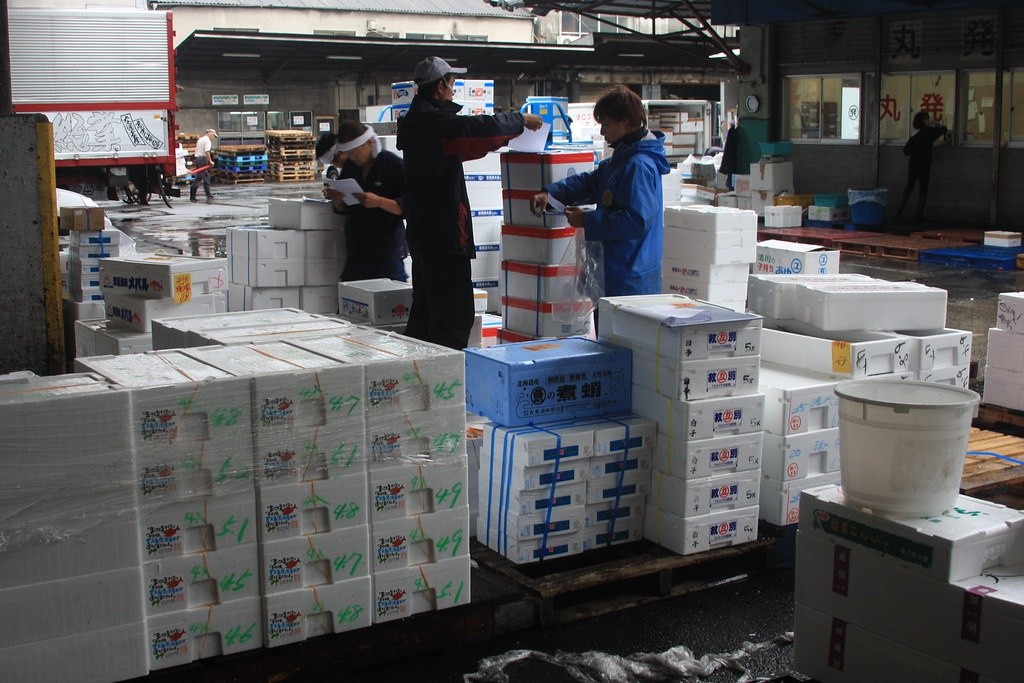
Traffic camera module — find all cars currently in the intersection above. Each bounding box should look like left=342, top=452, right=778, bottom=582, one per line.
left=321, top=163, right=342, bottom=182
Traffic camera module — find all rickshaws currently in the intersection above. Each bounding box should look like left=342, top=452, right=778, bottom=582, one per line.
left=117, top=164, right=214, bottom=204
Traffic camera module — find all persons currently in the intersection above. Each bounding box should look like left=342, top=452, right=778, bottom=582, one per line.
left=189, top=128, right=219, bottom=203
left=314, top=120, right=409, bottom=314
left=529, top=84, right=672, bottom=342
left=396, top=56, right=542, bottom=350
left=892, top=112, right=948, bottom=220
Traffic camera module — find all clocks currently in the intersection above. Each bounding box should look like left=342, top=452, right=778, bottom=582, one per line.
left=745, top=95, right=760, bottom=113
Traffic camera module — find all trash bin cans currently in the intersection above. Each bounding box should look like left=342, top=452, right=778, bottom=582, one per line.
left=850, top=188, right=888, bottom=225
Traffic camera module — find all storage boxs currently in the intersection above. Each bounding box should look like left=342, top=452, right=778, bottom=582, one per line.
left=0, top=79, right=1023, bottom=683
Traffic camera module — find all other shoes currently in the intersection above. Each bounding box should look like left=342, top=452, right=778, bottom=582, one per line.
left=191, top=198, right=200, bottom=202
left=208, top=194, right=216, bottom=199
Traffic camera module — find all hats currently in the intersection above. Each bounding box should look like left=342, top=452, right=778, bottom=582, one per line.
left=207, top=129, right=218, bottom=138
left=414, top=56, right=467, bottom=86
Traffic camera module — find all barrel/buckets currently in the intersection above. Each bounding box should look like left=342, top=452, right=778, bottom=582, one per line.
left=833, top=378, right=980, bottom=518
